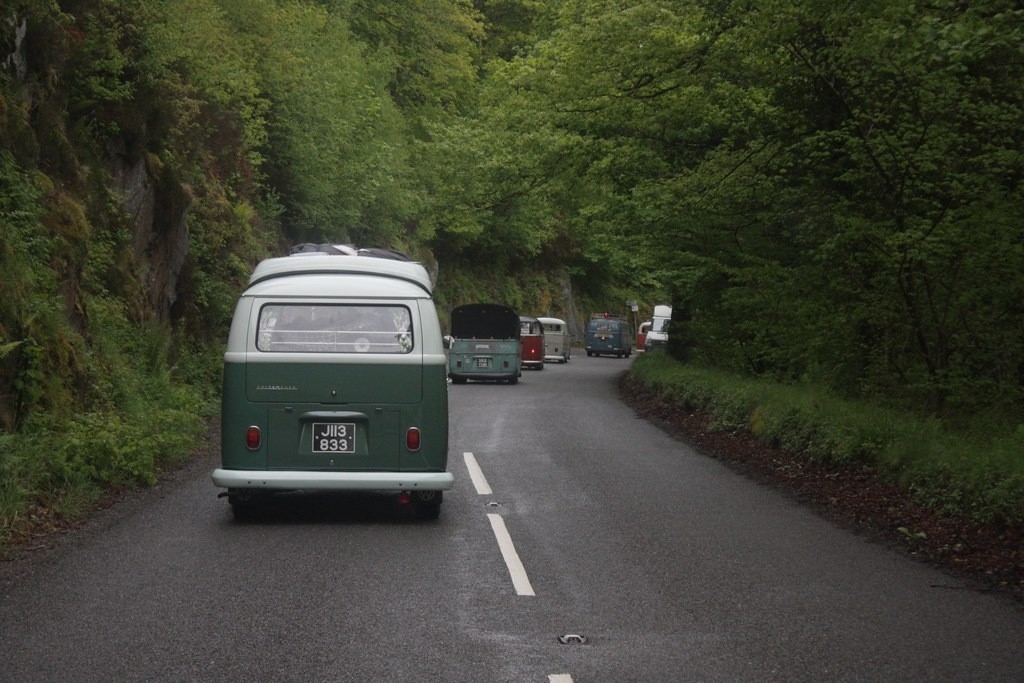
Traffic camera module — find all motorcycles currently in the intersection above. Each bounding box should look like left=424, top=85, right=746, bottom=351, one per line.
left=211, top=241, right=456, bottom=519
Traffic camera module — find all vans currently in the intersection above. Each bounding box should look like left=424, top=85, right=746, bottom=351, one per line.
left=516, top=317, right=547, bottom=371
left=583, top=313, right=634, bottom=358
left=637, top=304, right=674, bottom=353
left=534, top=317, right=572, bottom=365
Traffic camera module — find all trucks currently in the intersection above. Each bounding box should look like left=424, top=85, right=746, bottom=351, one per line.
left=443, top=302, right=524, bottom=386
